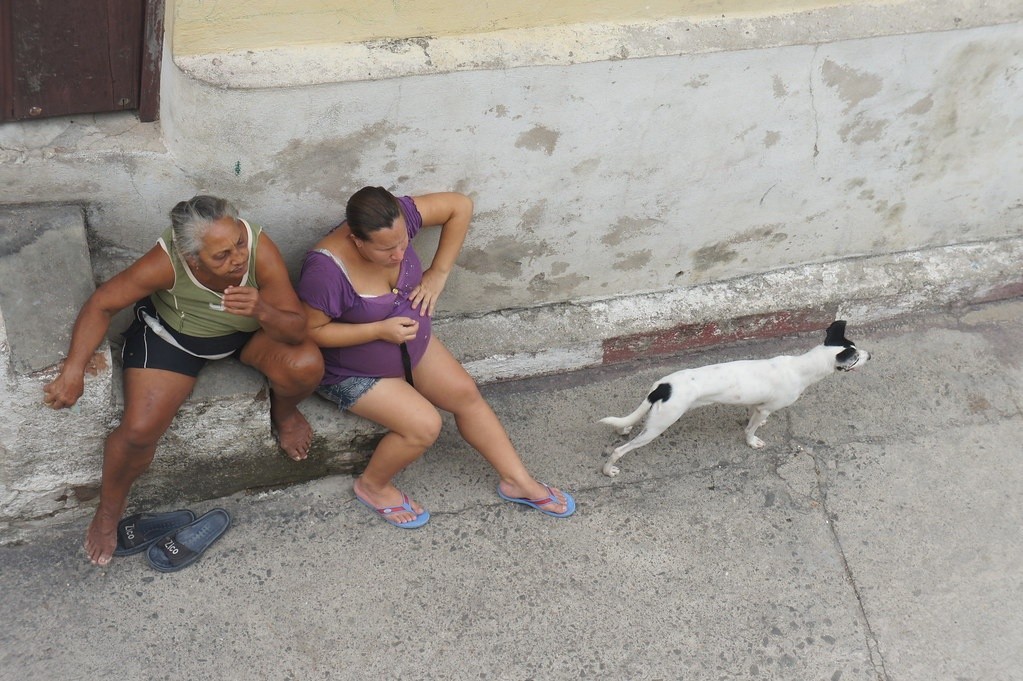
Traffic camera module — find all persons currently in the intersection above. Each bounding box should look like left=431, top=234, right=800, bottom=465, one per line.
left=43, top=195, right=325, bottom=568
left=298, top=185, right=575, bottom=530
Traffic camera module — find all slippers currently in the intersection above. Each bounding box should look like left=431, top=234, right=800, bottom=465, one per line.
left=146, top=508, right=230, bottom=572
left=497, top=476, right=576, bottom=517
left=356, top=485, right=430, bottom=529
left=113, top=509, right=195, bottom=556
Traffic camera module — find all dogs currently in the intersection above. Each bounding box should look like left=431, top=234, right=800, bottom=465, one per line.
left=594, top=319, right=871, bottom=477
